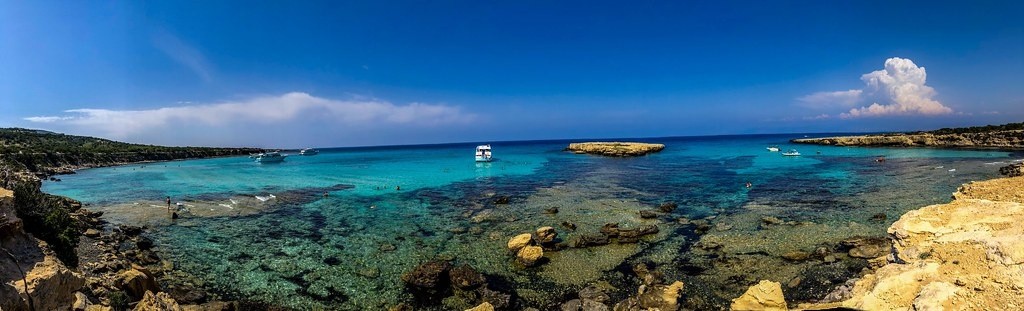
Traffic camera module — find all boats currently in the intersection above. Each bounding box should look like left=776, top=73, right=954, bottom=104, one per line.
left=781, top=149, right=802, bottom=156
left=766, top=146, right=781, bottom=151
left=298, top=147, right=319, bottom=155
left=475, top=144, right=493, bottom=162
left=256, top=152, right=288, bottom=162
